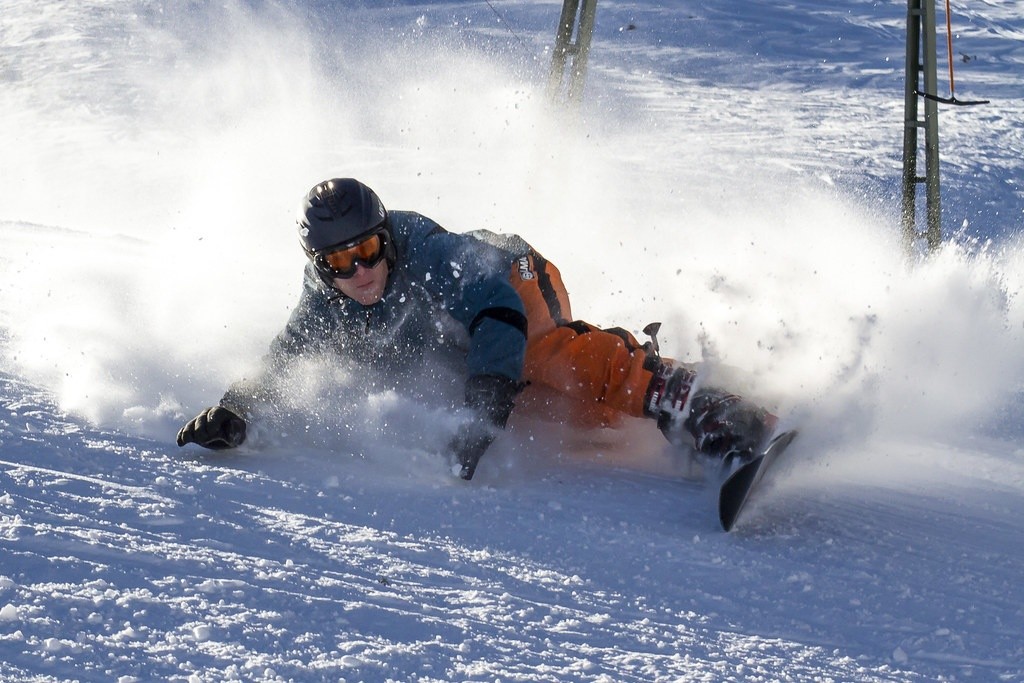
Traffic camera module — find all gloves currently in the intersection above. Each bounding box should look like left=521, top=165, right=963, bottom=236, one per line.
left=177, top=406, right=246, bottom=449
left=447, top=373, right=516, bottom=481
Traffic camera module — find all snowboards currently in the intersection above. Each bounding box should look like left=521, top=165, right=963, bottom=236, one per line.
left=718, top=354, right=886, bottom=535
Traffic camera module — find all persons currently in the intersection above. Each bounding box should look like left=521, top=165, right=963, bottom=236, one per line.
left=177, top=177, right=797, bottom=532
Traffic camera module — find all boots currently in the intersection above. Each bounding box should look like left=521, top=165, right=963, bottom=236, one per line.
left=645, top=359, right=780, bottom=487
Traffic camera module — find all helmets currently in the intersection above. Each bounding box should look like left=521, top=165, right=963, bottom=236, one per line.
left=296, top=178, right=396, bottom=284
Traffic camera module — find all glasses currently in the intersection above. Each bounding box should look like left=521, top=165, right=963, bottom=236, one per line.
left=298, top=208, right=391, bottom=279
